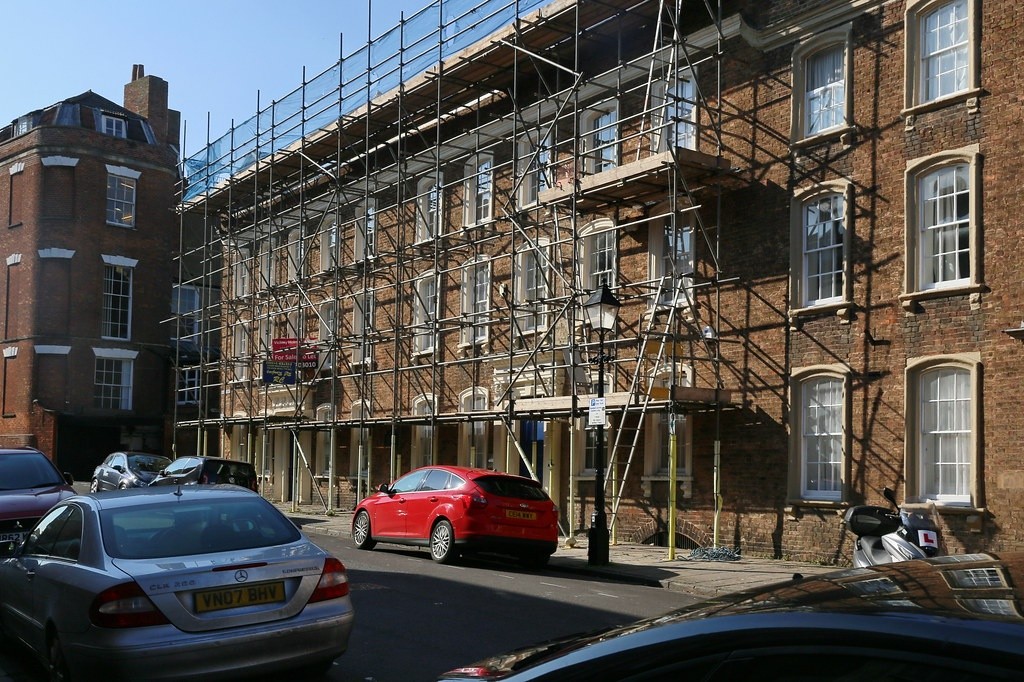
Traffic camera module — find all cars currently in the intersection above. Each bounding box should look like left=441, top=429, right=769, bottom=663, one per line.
left=0, top=443, right=78, bottom=561
left=432, top=553, right=989, bottom=681
left=350, top=464, right=559, bottom=572
left=0, top=482, right=356, bottom=681
left=89, top=451, right=172, bottom=493
left=147, top=455, right=257, bottom=493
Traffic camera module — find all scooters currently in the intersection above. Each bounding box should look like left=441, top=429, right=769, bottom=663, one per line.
left=851, top=485, right=943, bottom=569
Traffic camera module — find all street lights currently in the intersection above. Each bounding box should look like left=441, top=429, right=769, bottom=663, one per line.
left=581, top=277, right=625, bottom=564
